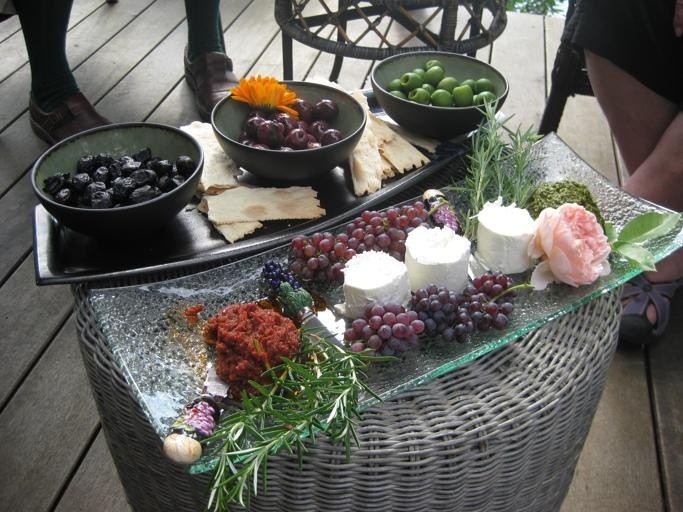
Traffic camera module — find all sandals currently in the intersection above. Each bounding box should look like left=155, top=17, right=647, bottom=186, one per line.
left=617, top=272, right=682, bottom=355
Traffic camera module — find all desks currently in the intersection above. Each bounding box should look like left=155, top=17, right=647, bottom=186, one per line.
left=68, top=138, right=625, bottom=512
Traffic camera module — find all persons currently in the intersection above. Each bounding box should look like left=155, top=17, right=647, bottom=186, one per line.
left=562, top=0, right=683, bottom=345
left=11, top=0, right=241, bottom=146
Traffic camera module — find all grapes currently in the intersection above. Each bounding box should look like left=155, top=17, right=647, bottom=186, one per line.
left=291, top=201, right=536, bottom=357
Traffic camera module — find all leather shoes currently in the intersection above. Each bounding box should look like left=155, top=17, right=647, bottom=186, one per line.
left=27, top=92, right=114, bottom=147
left=182, top=44, right=241, bottom=124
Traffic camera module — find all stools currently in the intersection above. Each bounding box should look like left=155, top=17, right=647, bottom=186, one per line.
left=538, top=0, right=601, bottom=139
left=272, top=0, right=513, bottom=84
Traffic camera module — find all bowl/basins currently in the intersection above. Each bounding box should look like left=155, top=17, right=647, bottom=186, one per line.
left=209, top=78, right=365, bottom=182
left=29, top=121, right=204, bottom=238
left=369, top=49, right=509, bottom=137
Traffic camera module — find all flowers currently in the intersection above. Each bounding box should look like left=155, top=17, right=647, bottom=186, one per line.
left=522, top=199, right=681, bottom=298
left=223, top=75, right=300, bottom=119
